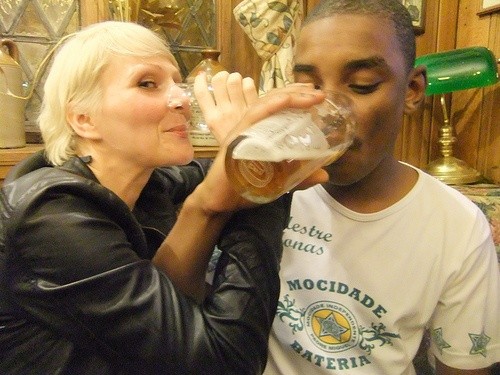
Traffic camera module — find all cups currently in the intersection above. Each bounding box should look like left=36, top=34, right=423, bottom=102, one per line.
left=223, top=90, right=360, bottom=204
left=162, top=83, right=216, bottom=134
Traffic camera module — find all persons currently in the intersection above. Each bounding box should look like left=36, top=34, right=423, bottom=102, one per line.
left=0, top=21, right=282, bottom=375
left=149, top=0, right=500, bottom=375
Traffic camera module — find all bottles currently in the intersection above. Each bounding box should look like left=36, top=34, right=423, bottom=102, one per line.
left=184, top=50, right=227, bottom=134
left=1, top=38, right=25, bottom=148
